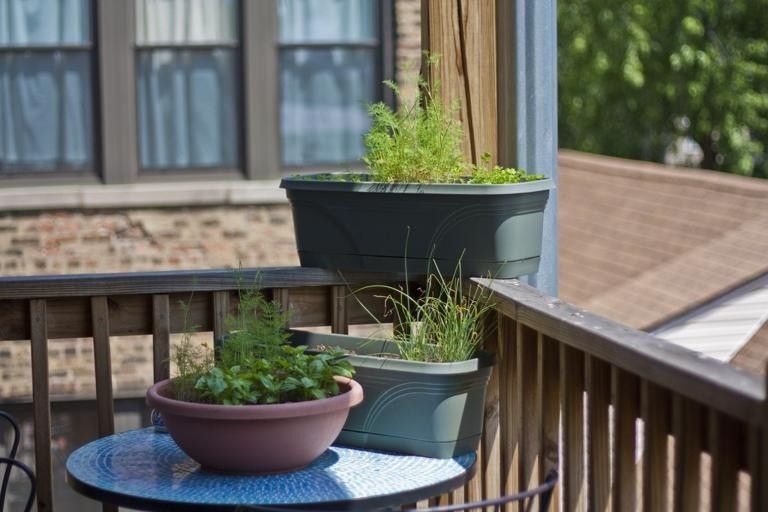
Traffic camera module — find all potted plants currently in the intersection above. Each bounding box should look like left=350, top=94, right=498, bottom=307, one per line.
left=214, top=223, right=507, bottom=459
left=279, top=50, right=554, bottom=278
left=146, top=260, right=365, bottom=467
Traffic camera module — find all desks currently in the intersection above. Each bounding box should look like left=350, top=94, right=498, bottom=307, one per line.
left=65, top=425, right=477, bottom=512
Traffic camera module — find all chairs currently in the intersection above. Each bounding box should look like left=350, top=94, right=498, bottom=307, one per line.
left=0, top=410, right=37, bottom=512
